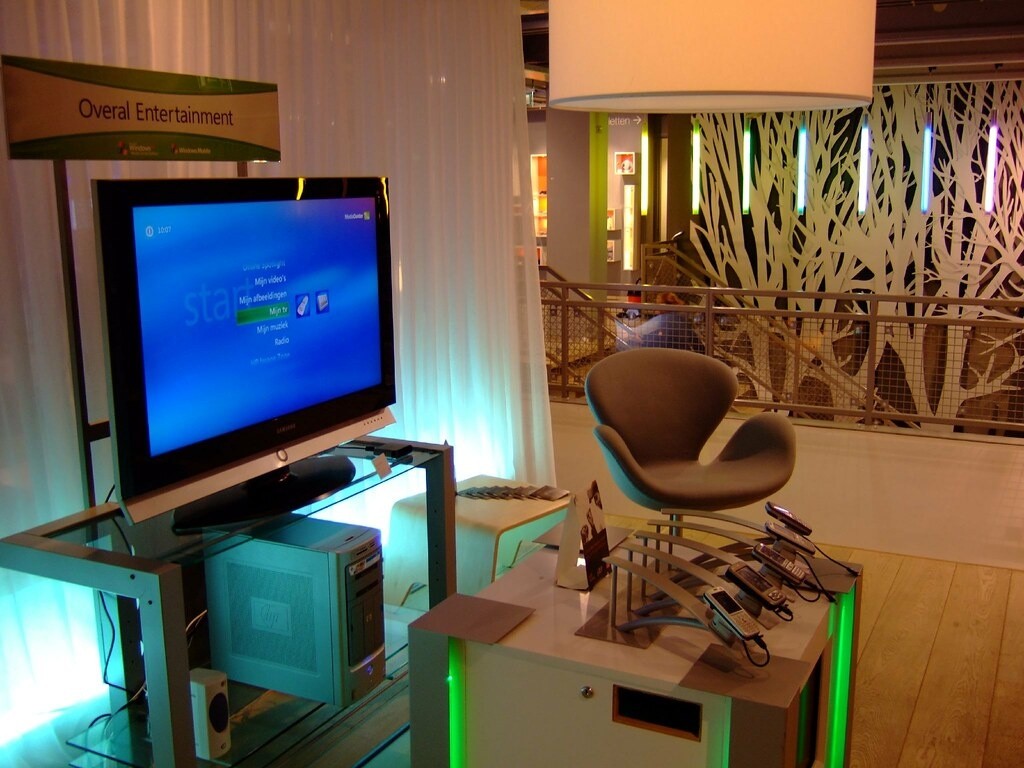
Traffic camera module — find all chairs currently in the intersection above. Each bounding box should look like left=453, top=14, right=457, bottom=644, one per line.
left=584, top=346, right=799, bottom=537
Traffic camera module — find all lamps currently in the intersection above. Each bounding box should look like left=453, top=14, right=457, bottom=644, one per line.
left=548, top=0, right=876, bottom=115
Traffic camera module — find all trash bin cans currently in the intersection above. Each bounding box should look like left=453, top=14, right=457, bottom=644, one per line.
left=627, top=290, right=641, bottom=302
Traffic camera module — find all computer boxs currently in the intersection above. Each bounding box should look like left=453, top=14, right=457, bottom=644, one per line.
left=201, top=520, right=387, bottom=706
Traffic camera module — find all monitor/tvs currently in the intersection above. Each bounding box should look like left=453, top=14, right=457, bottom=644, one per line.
left=89, top=175, right=396, bottom=533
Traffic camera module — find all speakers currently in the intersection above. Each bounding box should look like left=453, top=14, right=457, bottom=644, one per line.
left=191, top=667, right=231, bottom=761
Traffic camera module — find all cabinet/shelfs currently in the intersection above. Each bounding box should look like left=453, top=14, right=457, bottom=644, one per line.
left=0, top=431, right=454, bottom=768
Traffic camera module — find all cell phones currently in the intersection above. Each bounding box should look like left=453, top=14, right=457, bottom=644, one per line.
left=704, top=585, right=760, bottom=640
left=726, top=561, right=787, bottom=610
left=749, top=543, right=807, bottom=586
left=764, top=502, right=813, bottom=535
left=762, top=520, right=816, bottom=556
